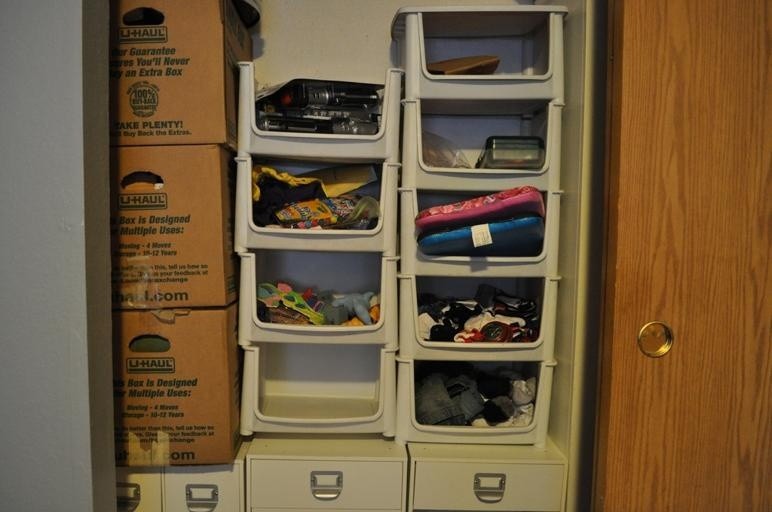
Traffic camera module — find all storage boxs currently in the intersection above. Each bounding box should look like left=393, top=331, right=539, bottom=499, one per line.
left=109, top=0, right=253, bottom=149
left=109, top=145, right=237, bottom=310
left=114, top=300, right=242, bottom=467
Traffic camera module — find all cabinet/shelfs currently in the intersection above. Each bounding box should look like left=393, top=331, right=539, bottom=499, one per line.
left=114, top=441, right=250, bottom=512
left=407, top=444, right=583, bottom=511
left=244, top=434, right=407, bottom=512
left=233, top=61, right=396, bottom=438
left=397, top=0, right=566, bottom=448
